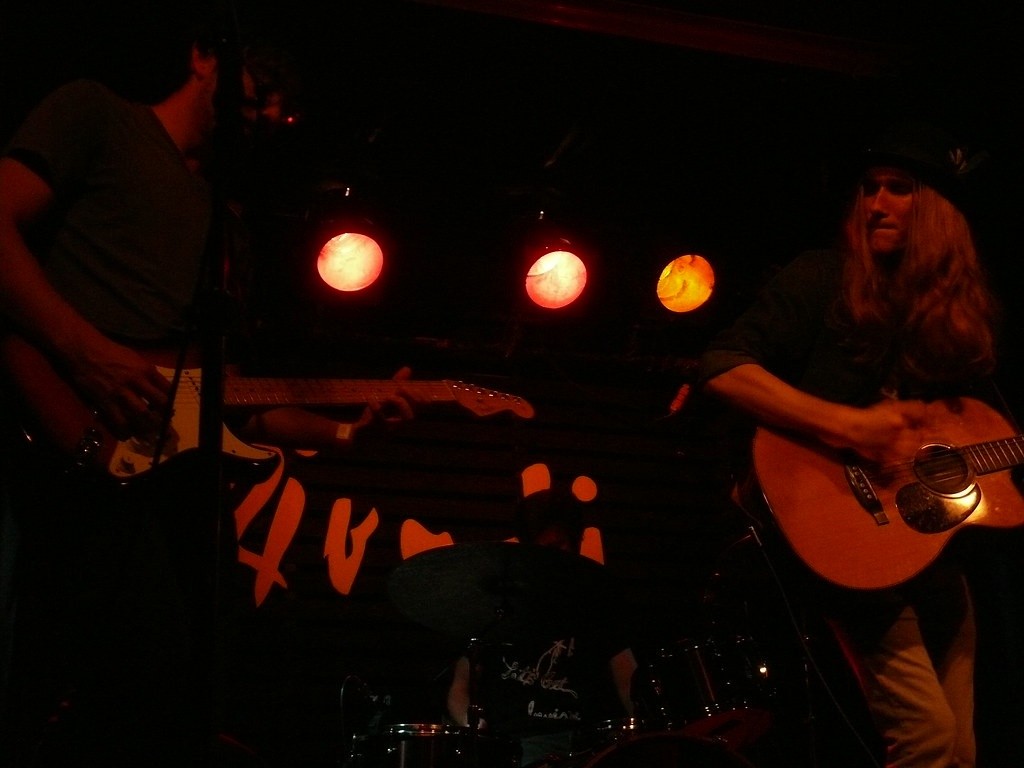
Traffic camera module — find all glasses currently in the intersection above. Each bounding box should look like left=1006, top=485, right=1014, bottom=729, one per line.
left=244, top=66, right=305, bottom=126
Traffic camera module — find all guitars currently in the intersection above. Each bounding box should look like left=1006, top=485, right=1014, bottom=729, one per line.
left=741, top=399, right=1024, bottom=614
left=106, top=352, right=540, bottom=484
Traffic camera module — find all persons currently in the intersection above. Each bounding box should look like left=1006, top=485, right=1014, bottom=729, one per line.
left=1, top=0, right=419, bottom=765
left=699, top=134, right=1000, bottom=768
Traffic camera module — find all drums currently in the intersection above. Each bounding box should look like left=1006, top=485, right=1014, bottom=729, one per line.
left=361, top=723, right=525, bottom=768
left=546, top=720, right=740, bottom=768
left=682, top=706, right=780, bottom=768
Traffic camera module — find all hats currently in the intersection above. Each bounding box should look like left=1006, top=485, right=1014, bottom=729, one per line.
left=853, top=119, right=976, bottom=194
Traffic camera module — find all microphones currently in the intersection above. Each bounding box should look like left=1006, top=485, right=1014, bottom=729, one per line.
left=349, top=675, right=380, bottom=705
